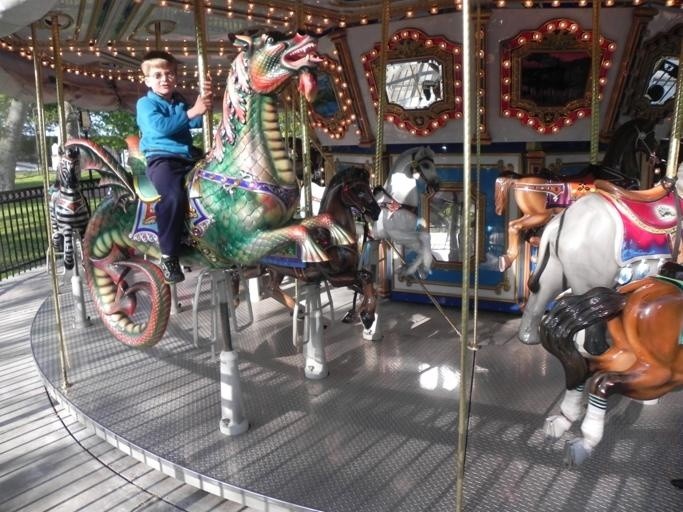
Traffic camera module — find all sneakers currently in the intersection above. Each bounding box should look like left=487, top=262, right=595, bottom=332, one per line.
left=159, top=255, right=185, bottom=285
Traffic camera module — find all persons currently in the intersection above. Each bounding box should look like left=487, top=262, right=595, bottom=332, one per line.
left=133, top=48, right=214, bottom=287
left=652, top=154, right=666, bottom=183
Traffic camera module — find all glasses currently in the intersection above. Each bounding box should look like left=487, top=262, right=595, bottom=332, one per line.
left=145, top=70, right=177, bottom=79
